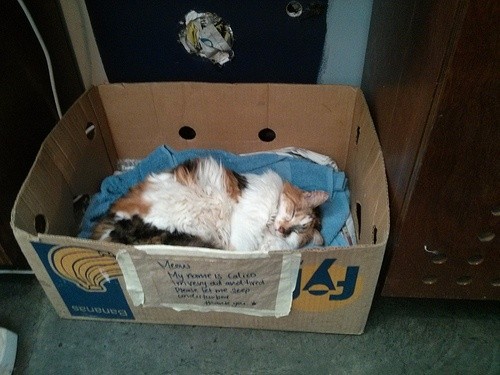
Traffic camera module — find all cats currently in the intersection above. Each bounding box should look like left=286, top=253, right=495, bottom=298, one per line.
left=87, top=153, right=330, bottom=252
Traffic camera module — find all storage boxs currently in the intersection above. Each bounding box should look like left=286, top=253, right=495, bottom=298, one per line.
left=10, top=83, right=392, bottom=338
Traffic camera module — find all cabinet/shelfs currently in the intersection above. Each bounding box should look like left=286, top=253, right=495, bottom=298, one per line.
left=362, top=0, right=500, bottom=315
